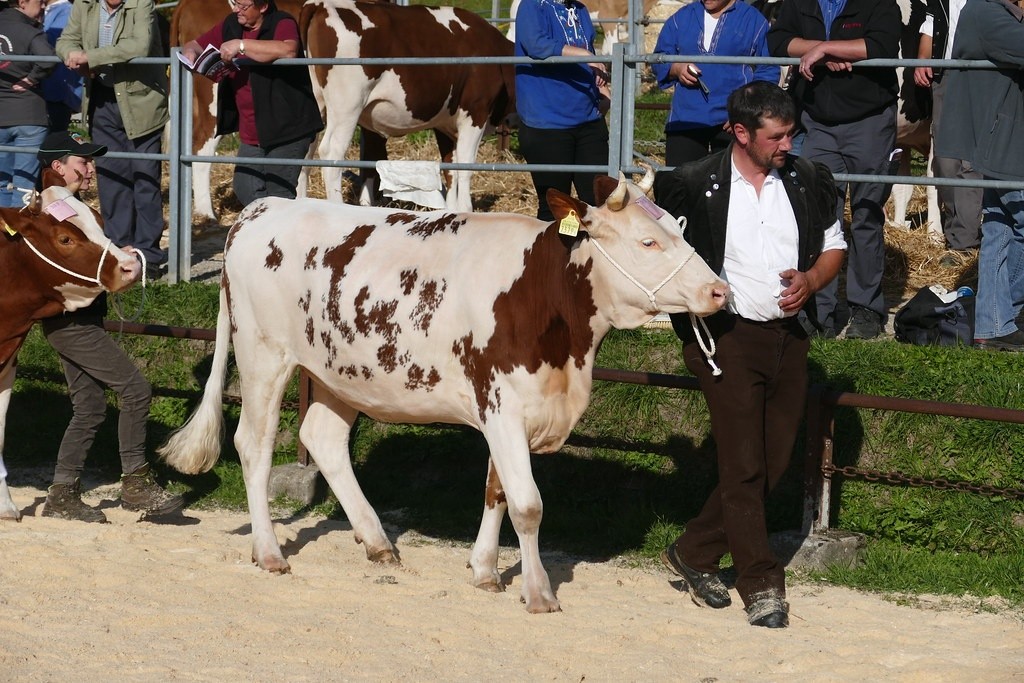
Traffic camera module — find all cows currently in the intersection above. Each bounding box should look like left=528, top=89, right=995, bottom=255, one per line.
left=0, top=166, right=142, bottom=524
left=892, top=0, right=944, bottom=247
left=155, top=160, right=733, bottom=612
left=163, top=1, right=310, bottom=224
left=504, top=0, right=663, bottom=72
left=298, top=1, right=520, bottom=216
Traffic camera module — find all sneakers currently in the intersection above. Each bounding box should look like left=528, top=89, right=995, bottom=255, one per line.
left=744, top=595, right=790, bottom=629
left=42, top=478, right=106, bottom=523
left=845, top=305, right=886, bottom=340
left=660, top=544, right=732, bottom=610
left=120, top=461, right=184, bottom=516
left=800, top=317, right=837, bottom=339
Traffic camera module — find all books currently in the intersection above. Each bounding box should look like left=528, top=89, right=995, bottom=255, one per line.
left=174, top=43, right=240, bottom=83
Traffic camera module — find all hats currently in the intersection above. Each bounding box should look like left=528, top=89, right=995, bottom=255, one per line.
left=36, top=131, right=109, bottom=168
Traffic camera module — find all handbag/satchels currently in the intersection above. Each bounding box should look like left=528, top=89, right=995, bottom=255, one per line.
left=894, top=285, right=977, bottom=347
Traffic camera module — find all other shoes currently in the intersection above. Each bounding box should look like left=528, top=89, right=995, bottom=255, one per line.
left=973, top=324, right=1024, bottom=351
left=939, top=249, right=970, bottom=266
left=140, top=262, right=161, bottom=281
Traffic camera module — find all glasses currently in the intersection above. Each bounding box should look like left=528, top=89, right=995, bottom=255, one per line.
left=229, top=0, right=254, bottom=11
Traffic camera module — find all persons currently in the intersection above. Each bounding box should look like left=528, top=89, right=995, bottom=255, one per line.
left=515, top=0, right=611, bottom=221
left=0, top=0, right=326, bottom=278
left=652, top=0, right=1024, bottom=350
left=39, top=131, right=185, bottom=523
left=659, top=79, right=849, bottom=627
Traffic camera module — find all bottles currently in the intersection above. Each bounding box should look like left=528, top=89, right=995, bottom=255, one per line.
left=956, top=286, right=975, bottom=298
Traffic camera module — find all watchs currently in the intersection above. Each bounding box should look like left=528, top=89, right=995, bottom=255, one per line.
left=239, top=38, right=246, bottom=58
